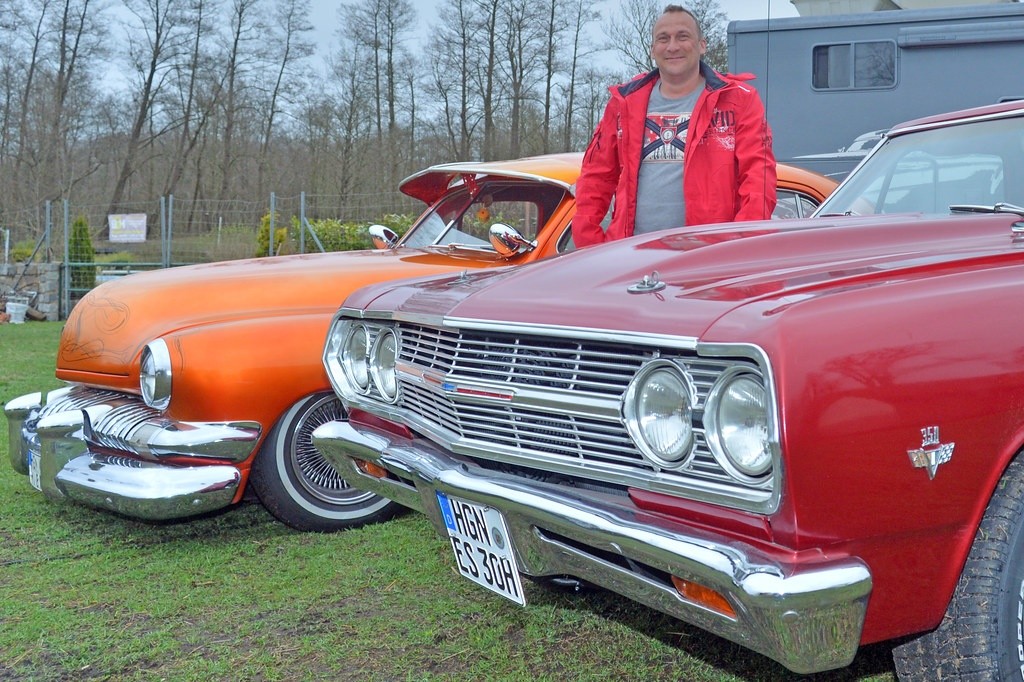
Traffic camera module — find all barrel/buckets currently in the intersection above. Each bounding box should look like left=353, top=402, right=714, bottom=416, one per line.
left=4, top=302, right=27, bottom=324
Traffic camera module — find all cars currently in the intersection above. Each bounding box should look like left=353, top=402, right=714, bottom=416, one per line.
left=4, top=151, right=840, bottom=534
left=310, top=95, right=1022, bottom=682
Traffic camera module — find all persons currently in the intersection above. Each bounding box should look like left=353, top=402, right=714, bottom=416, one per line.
left=570, top=3, right=777, bottom=251
